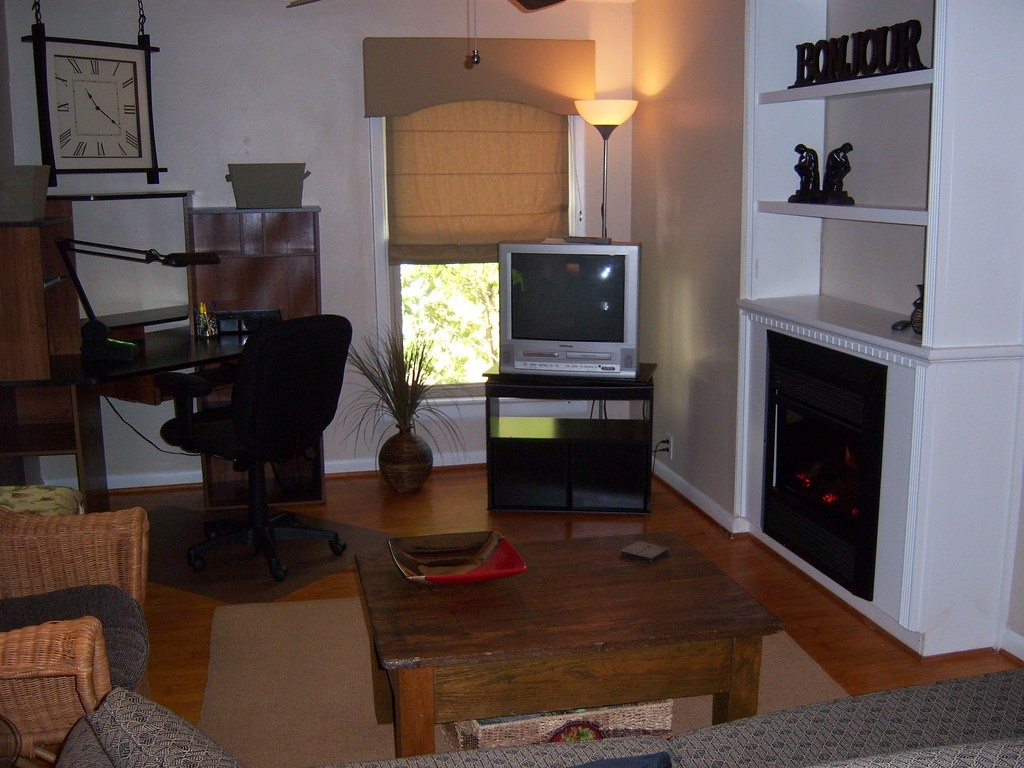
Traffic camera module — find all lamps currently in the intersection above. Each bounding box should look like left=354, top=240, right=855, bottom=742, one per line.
left=573, top=99, right=639, bottom=237
left=56, top=237, right=221, bottom=380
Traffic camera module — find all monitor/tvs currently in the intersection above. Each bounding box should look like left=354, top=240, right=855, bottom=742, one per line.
left=498, top=239, right=642, bottom=378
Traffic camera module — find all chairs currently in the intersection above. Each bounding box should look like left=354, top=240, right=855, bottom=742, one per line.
left=158, top=313, right=345, bottom=582
left=0, top=504, right=150, bottom=767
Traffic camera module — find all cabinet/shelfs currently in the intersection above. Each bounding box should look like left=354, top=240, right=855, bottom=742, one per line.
left=0, top=197, right=84, bottom=380
left=761, top=331, right=888, bottom=599
left=0, top=381, right=110, bottom=510
left=188, top=206, right=324, bottom=511
left=482, top=364, right=657, bottom=515
left=744, top=0, right=1024, bottom=351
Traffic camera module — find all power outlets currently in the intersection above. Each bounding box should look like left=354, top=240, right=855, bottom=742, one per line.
left=663, top=433, right=672, bottom=460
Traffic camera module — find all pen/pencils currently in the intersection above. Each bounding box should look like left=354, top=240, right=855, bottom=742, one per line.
left=194, top=302, right=216, bottom=336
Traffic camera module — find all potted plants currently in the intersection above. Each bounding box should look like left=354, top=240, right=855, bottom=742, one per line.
left=334, top=325, right=473, bottom=494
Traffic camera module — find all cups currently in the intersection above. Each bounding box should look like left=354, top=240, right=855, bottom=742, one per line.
left=194, top=313, right=218, bottom=336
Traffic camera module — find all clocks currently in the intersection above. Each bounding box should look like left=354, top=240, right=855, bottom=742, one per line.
left=22, top=23, right=169, bottom=186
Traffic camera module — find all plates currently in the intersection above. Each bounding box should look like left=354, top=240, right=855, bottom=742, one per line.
left=388, top=530, right=528, bottom=586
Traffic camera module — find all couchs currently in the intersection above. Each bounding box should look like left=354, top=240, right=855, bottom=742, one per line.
left=53, top=668, right=1024, bottom=768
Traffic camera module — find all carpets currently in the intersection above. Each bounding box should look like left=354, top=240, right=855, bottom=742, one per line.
left=196, top=597, right=848, bottom=768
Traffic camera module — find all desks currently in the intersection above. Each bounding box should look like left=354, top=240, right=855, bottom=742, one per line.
left=354, top=534, right=783, bottom=758
